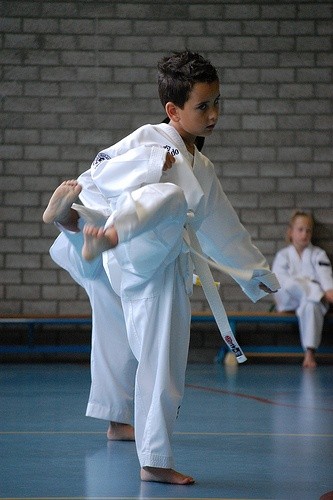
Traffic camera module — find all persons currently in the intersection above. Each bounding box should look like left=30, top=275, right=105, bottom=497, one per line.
left=43, top=117, right=206, bottom=442
left=272, top=211, right=333, bottom=367
left=81, top=52, right=280, bottom=485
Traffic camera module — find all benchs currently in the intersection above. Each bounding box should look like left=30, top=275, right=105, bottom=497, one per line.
left=0, top=314, right=333, bottom=359
